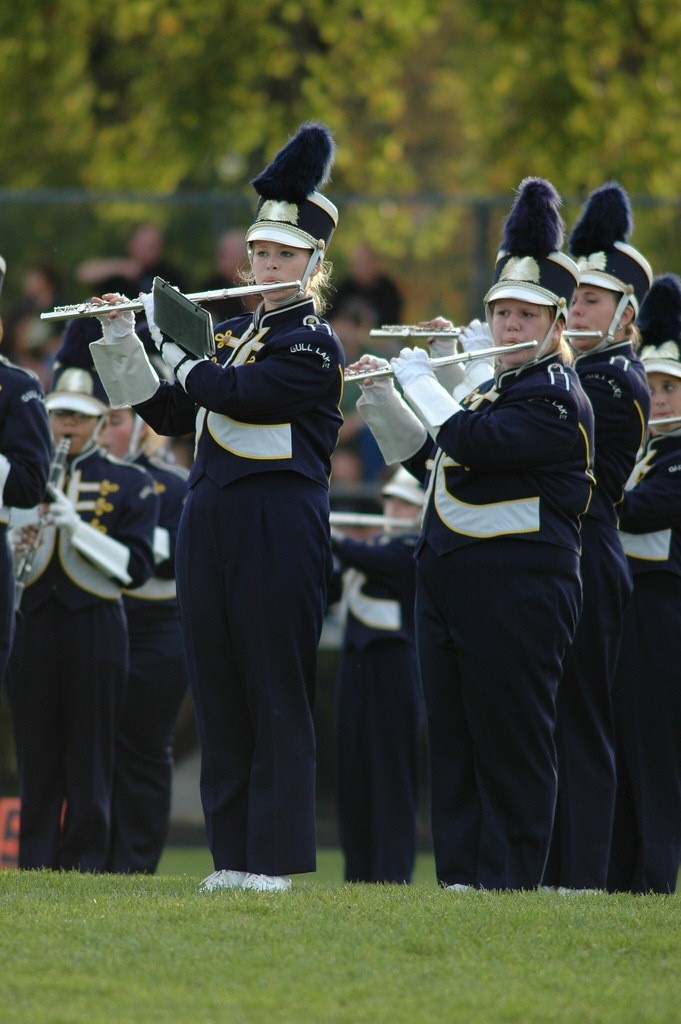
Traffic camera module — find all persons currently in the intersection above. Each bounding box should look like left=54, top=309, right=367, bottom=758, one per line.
left=0, top=184, right=681, bottom=875
left=345, top=177, right=593, bottom=889
left=91, top=126, right=347, bottom=892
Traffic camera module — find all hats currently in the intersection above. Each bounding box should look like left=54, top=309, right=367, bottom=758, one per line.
left=483, top=175, right=580, bottom=325
left=381, top=465, right=425, bottom=506
left=245, top=123, right=339, bottom=260
left=636, top=275, right=680, bottom=378
left=568, top=184, right=653, bottom=321
left=44, top=317, right=111, bottom=416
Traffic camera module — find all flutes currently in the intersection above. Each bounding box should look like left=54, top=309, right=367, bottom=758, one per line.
left=39, top=279, right=302, bottom=324
left=13, top=434, right=72, bottom=613
left=647, top=415, right=681, bottom=426
left=344, top=339, right=539, bottom=382
left=328, top=510, right=418, bottom=528
left=368, top=324, right=604, bottom=341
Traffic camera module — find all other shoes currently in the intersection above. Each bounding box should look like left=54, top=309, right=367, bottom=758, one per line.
left=201, top=869, right=244, bottom=891
left=241, top=871, right=293, bottom=892
